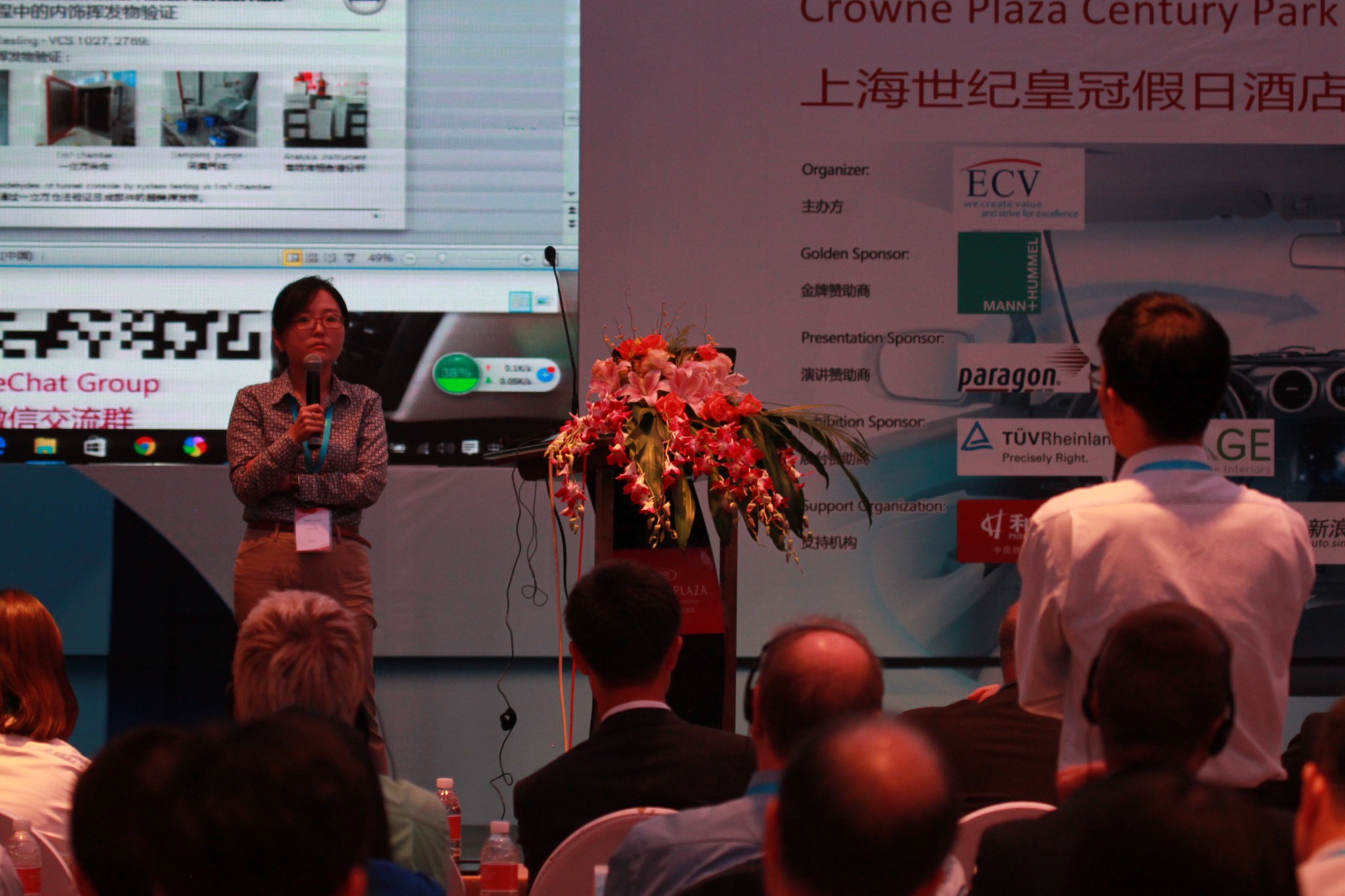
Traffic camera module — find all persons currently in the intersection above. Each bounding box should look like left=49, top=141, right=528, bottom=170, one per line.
left=512, top=561, right=757, bottom=896
left=603, top=617, right=886, bottom=896
left=1284, top=695, right=1345, bottom=896
left=967, top=601, right=1297, bottom=896
left=892, top=605, right=1075, bottom=821
left=67, top=589, right=452, bottom=896
left=1014, top=292, right=1316, bottom=811
left=758, top=720, right=963, bottom=896
left=225, top=277, right=389, bottom=723
left=0, top=590, right=95, bottom=896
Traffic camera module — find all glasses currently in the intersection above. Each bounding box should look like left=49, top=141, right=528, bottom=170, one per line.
left=293, top=315, right=345, bottom=331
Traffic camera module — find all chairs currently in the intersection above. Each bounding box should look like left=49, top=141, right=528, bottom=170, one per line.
left=528, top=805, right=680, bottom=896
left=952, top=796, right=1061, bottom=877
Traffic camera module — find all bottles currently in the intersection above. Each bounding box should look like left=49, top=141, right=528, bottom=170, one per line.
left=6, top=819, right=44, bottom=896
left=480, top=820, right=520, bottom=896
left=434, top=777, right=462, bottom=876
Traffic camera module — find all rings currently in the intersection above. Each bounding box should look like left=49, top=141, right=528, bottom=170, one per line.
left=311, top=412, right=315, bottom=418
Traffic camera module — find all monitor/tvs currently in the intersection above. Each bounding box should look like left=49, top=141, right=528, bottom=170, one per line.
left=611, top=339, right=736, bottom=402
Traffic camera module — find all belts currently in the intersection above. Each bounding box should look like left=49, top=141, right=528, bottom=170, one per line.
left=246, top=521, right=372, bottom=549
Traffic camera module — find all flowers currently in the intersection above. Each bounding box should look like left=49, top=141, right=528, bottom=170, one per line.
left=540, top=297, right=881, bottom=566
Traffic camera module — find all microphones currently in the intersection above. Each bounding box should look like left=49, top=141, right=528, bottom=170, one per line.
left=544, top=245, right=578, bottom=413
left=301, top=354, right=324, bottom=438
